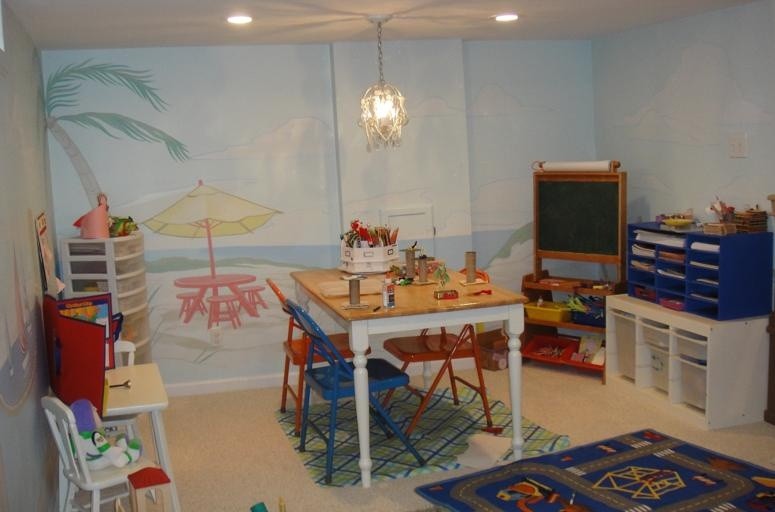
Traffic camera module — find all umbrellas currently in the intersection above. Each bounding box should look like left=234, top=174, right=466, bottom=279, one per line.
left=142, top=178, right=284, bottom=239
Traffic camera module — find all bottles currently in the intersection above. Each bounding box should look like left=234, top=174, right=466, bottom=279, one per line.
left=382, top=272, right=396, bottom=310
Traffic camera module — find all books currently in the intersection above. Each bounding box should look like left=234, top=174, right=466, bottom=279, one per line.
left=629, top=228, right=720, bottom=304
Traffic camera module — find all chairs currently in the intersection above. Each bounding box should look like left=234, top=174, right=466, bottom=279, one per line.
left=375, top=267, right=492, bottom=438
left=39, top=396, right=159, bottom=512
left=287, top=301, right=426, bottom=487
left=102, top=339, right=142, bottom=441
left=266, top=281, right=374, bottom=433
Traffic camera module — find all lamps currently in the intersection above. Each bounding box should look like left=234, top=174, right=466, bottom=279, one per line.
left=355, top=14, right=410, bottom=153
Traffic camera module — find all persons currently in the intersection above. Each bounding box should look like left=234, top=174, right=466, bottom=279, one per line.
left=66, top=399, right=143, bottom=471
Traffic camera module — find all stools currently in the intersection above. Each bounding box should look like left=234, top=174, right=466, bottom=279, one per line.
left=175, top=286, right=269, bottom=330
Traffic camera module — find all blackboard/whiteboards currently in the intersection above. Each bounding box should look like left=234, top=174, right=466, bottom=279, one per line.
left=533, top=173, right=627, bottom=259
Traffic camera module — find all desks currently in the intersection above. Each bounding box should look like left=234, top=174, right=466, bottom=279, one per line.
left=174, top=274, right=259, bottom=323
left=288, top=262, right=530, bottom=486
left=103, top=362, right=180, bottom=511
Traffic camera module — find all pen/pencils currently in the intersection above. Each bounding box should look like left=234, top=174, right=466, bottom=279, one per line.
left=373, top=306, right=381, bottom=311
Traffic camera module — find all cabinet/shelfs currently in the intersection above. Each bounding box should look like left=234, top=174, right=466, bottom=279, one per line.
left=59, top=231, right=151, bottom=366
left=519, top=269, right=626, bottom=385
left=605, top=219, right=774, bottom=434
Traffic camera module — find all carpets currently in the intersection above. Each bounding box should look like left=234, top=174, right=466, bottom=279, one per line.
left=274, top=385, right=570, bottom=489
left=415, top=426, right=775, bottom=512
